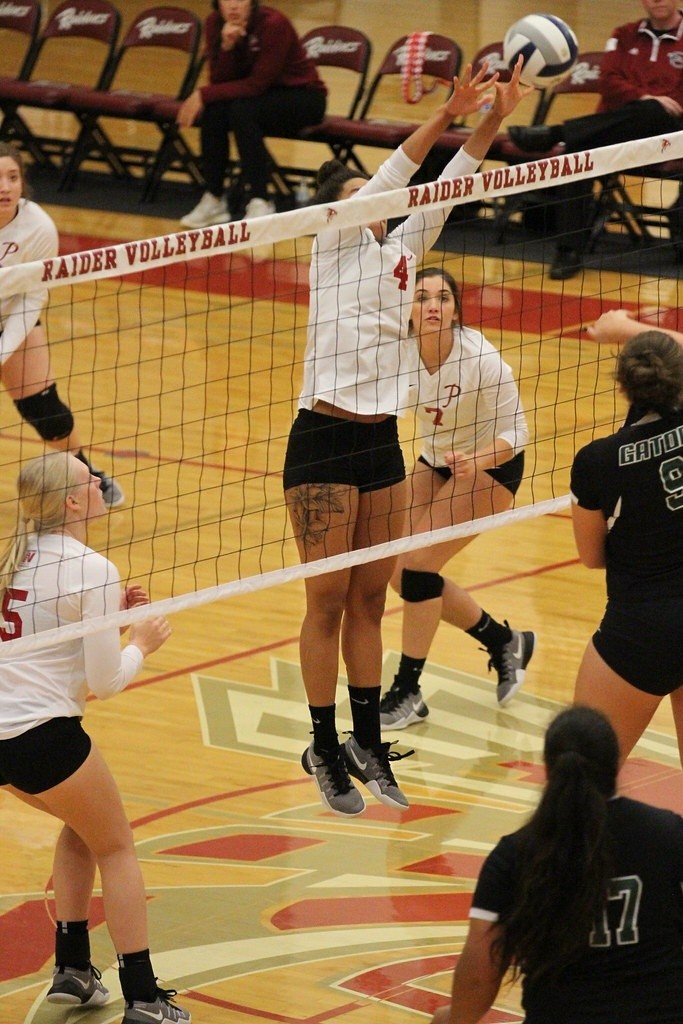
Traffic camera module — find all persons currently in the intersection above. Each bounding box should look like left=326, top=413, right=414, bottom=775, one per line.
left=569, top=309, right=683, bottom=772
left=508, top=0, right=683, bottom=280
left=283, top=52, right=538, bottom=818
left=178, top=0, right=327, bottom=227
left=429, top=706, right=683, bottom=1024
left=0, top=142, right=124, bottom=507
left=0, top=454, right=193, bottom=1024
left=378, top=268, right=535, bottom=732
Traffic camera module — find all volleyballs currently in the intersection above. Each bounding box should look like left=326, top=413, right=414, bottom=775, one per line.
left=504, top=12, right=579, bottom=86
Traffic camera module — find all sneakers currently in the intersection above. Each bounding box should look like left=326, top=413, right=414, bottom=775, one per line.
left=340, top=730, right=418, bottom=811
left=97, top=471, right=124, bottom=509
left=376, top=675, right=431, bottom=730
left=122, top=990, right=193, bottom=1024
left=244, top=197, right=277, bottom=219
left=479, top=619, right=540, bottom=705
left=300, top=740, right=369, bottom=817
left=44, top=962, right=116, bottom=1009
left=178, top=192, right=234, bottom=231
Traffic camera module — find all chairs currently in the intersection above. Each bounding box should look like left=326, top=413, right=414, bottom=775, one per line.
left=1, top=1, right=683, bottom=253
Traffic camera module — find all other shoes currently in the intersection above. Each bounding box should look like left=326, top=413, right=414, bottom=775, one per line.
left=506, top=123, right=556, bottom=155
left=548, top=251, right=584, bottom=280
